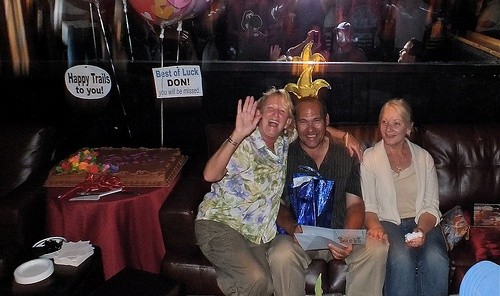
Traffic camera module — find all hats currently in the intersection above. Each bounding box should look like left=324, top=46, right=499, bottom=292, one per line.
left=333, top=21, right=352, bottom=32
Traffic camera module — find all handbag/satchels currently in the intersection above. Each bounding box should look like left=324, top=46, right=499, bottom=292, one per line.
left=440, top=206, right=471, bottom=251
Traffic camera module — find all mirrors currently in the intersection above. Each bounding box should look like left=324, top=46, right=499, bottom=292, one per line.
left=20, top=0, right=500, bottom=75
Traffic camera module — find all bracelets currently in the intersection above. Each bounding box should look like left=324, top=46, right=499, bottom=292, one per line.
left=228, top=137, right=238, bottom=147
left=342, top=132, right=347, bottom=141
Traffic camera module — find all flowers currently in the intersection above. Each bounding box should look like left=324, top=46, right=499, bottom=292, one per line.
left=56, top=149, right=119, bottom=174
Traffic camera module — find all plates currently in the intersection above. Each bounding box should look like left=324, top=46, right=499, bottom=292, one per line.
left=14, top=259, right=54, bottom=284
left=33, top=237, right=67, bottom=257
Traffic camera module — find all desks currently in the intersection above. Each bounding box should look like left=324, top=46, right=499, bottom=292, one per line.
left=46, top=170, right=184, bottom=283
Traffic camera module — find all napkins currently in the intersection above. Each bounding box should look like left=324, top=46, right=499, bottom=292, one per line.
left=39, top=238, right=95, bottom=269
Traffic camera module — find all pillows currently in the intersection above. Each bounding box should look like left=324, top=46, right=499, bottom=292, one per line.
left=440, top=205, right=471, bottom=252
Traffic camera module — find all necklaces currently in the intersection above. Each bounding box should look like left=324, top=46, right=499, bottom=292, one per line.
left=387, top=144, right=404, bottom=173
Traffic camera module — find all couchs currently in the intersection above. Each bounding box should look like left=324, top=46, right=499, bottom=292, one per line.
left=160, top=121, right=500, bottom=296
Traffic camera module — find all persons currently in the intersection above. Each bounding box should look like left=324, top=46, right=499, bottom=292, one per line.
left=398, top=38, right=426, bottom=63
left=359, top=98, right=449, bottom=296
left=194, top=88, right=364, bottom=296
left=266, top=96, right=390, bottom=296
left=424, top=0, right=450, bottom=58
left=476, top=0, right=500, bottom=39
left=269, top=21, right=367, bottom=62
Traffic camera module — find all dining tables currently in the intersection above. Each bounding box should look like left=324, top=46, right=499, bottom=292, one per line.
left=0, top=243, right=109, bottom=296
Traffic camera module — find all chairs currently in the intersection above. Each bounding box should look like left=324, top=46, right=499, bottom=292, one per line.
left=423, top=25, right=448, bottom=61
left=351, top=26, right=378, bottom=62
left=319, top=26, right=334, bottom=54
